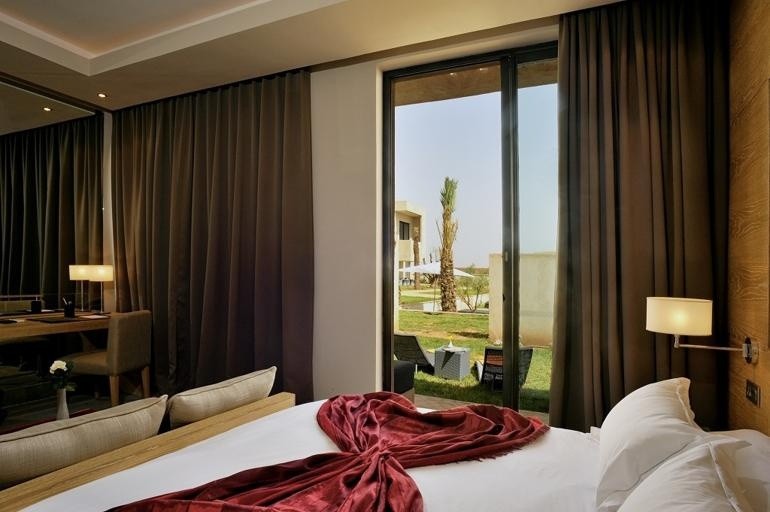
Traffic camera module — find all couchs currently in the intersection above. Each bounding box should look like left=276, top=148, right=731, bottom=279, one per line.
left=0, top=389, right=297, bottom=512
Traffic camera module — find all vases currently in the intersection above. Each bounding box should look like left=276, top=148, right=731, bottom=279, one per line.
left=447, top=340, right=453, bottom=347
left=55, top=387, right=69, bottom=419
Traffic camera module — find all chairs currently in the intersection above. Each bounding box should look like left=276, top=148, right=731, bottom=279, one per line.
left=0, top=300, right=44, bottom=311
left=62, top=309, right=152, bottom=407
left=394, top=334, right=434, bottom=374
left=474, top=345, right=533, bottom=394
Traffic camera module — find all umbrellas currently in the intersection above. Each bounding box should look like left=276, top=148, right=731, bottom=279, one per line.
left=398, top=261, right=473, bottom=311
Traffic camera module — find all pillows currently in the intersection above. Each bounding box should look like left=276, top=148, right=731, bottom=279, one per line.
left=0, top=391, right=168, bottom=486
left=590, top=375, right=753, bottom=512
left=166, top=364, right=277, bottom=429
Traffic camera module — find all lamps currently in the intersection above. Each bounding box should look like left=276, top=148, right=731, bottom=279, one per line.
left=645, top=295, right=758, bottom=363
left=69, top=265, right=89, bottom=312
left=88, top=265, right=113, bottom=314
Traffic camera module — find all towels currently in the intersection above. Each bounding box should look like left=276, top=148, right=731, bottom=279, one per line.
left=108, top=390, right=550, bottom=512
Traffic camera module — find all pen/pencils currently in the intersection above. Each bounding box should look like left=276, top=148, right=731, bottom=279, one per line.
left=62, top=296, right=72, bottom=306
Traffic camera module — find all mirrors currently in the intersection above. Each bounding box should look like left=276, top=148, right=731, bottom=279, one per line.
left=0, top=71, right=104, bottom=317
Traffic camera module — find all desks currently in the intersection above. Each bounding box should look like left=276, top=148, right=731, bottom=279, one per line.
left=0, top=308, right=124, bottom=344
left=434, top=346, right=471, bottom=383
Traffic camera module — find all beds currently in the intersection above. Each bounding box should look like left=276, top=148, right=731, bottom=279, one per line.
left=18, top=396, right=770, bottom=512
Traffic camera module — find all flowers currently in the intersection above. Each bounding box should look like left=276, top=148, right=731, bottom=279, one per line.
left=448, top=335, right=454, bottom=340
left=49, top=358, right=76, bottom=391
